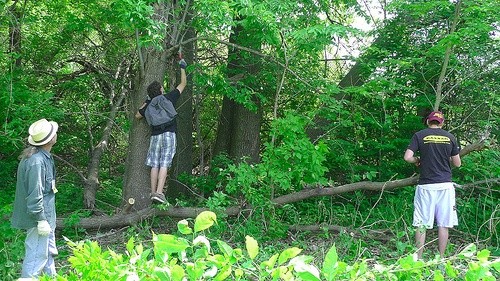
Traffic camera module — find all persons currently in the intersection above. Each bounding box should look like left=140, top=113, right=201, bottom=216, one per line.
left=10, top=118, right=58, bottom=281
left=134, top=58, right=187, bottom=205
left=404, top=111, right=461, bottom=260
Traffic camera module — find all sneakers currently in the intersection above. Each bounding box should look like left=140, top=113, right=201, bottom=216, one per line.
left=153, top=193, right=168, bottom=204
left=150, top=193, right=155, bottom=203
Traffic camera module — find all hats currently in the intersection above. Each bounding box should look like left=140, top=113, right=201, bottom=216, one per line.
left=429, top=111, right=445, bottom=124
left=28, top=119, right=58, bottom=145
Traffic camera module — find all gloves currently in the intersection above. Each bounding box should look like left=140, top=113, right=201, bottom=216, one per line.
left=179, top=60, right=186, bottom=68
left=37, top=220, right=52, bottom=236
